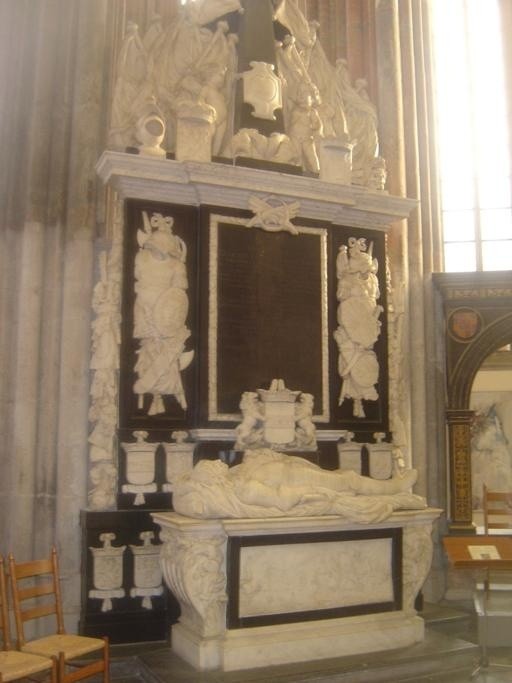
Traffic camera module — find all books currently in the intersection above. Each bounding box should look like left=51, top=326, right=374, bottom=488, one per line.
left=468, top=542, right=502, bottom=559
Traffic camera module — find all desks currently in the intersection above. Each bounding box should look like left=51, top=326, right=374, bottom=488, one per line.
left=441, top=533, right=510, bottom=667
left=148, top=506, right=444, bottom=672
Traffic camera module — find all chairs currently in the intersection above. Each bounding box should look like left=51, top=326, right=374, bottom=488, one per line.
left=484, top=482, right=512, bottom=592
left=0, top=556, right=60, bottom=683
left=8, top=545, right=112, bottom=683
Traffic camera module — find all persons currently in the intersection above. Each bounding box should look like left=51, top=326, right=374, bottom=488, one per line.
left=287, top=81, right=322, bottom=172
left=198, top=61, right=229, bottom=155
left=193, top=447, right=417, bottom=510
